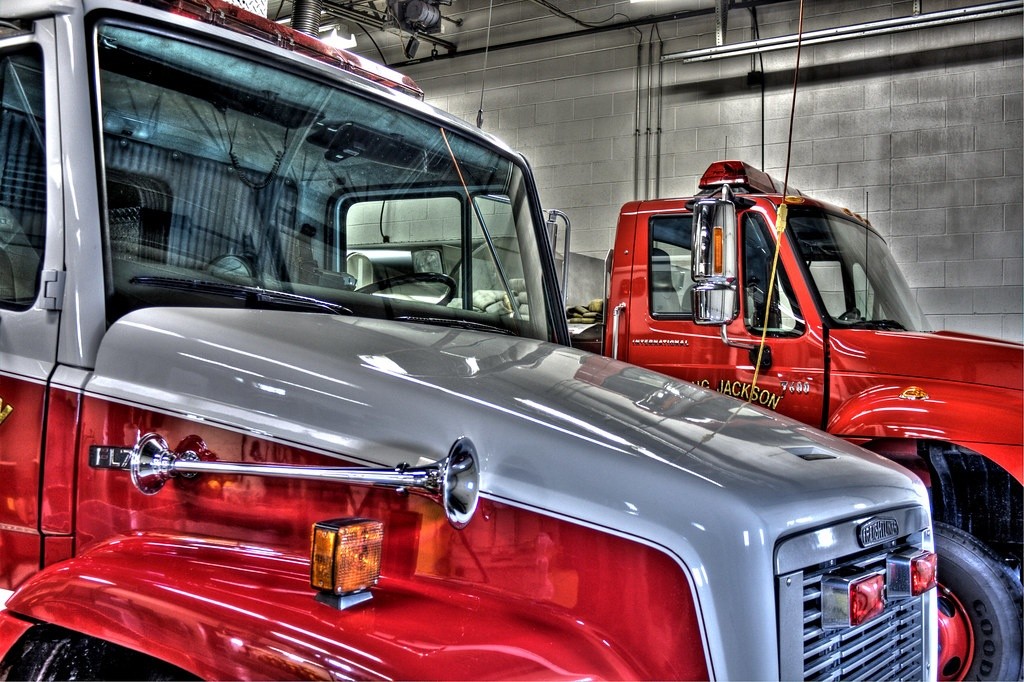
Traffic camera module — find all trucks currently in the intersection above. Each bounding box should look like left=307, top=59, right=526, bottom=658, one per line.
left=0, top=1, right=1022, bottom=682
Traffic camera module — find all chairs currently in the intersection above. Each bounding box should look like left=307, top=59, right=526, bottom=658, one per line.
left=652, top=247, right=680, bottom=312
